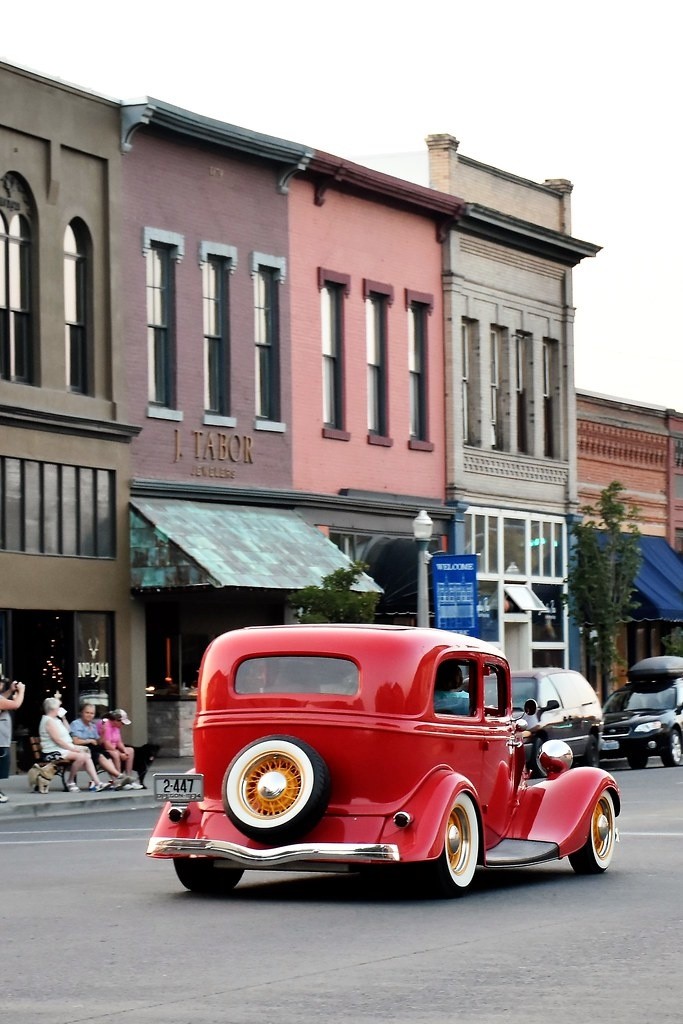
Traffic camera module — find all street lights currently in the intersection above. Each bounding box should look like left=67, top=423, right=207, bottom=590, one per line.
left=411, top=509, right=436, bottom=628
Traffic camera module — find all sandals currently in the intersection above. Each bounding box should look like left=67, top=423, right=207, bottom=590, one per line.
left=66, top=781, right=80, bottom=792
left=95, top=782, right=111, bottom=791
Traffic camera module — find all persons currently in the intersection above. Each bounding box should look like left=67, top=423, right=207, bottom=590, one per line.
left=0, top=675, right=144, bottom=803
left=436, top=664, right=463, bottom=692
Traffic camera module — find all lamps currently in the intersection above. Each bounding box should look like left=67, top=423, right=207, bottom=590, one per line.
left=543, top=600, right=557, bottom=620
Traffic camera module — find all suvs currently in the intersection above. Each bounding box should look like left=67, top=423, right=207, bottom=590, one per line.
left=461, top=666, right=604, bottom=777
left=599, top=656, right=683, bottom=770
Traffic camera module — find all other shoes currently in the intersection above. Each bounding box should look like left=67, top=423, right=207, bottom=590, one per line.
left=122, top=784, right=132, bottom=790
left=0, top=792, right=10, bottom=803
left=113, top=773, right=137, bottom=790
left=130, top=782, right=143, bottom=789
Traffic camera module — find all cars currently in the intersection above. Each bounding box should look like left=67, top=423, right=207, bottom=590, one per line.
left=143, top=621, right=621, bottom=899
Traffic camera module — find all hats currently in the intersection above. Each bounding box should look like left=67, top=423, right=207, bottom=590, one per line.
left=112, top=709, right=131, bottom=725
left=0, top=675, right=10, bottom=682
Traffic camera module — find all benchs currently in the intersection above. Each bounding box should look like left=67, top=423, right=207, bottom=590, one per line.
left=30, top=736, right=106, bottom=792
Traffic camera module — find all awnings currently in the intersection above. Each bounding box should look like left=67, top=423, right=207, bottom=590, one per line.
left=583, top=529, right=683, bottom=623
left=128, top=496, right=384, bottom=594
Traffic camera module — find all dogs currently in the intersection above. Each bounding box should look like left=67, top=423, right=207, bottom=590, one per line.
left=27, top=762, right=61, bottom=794
left=121, top=743, right=160, bottom=790
